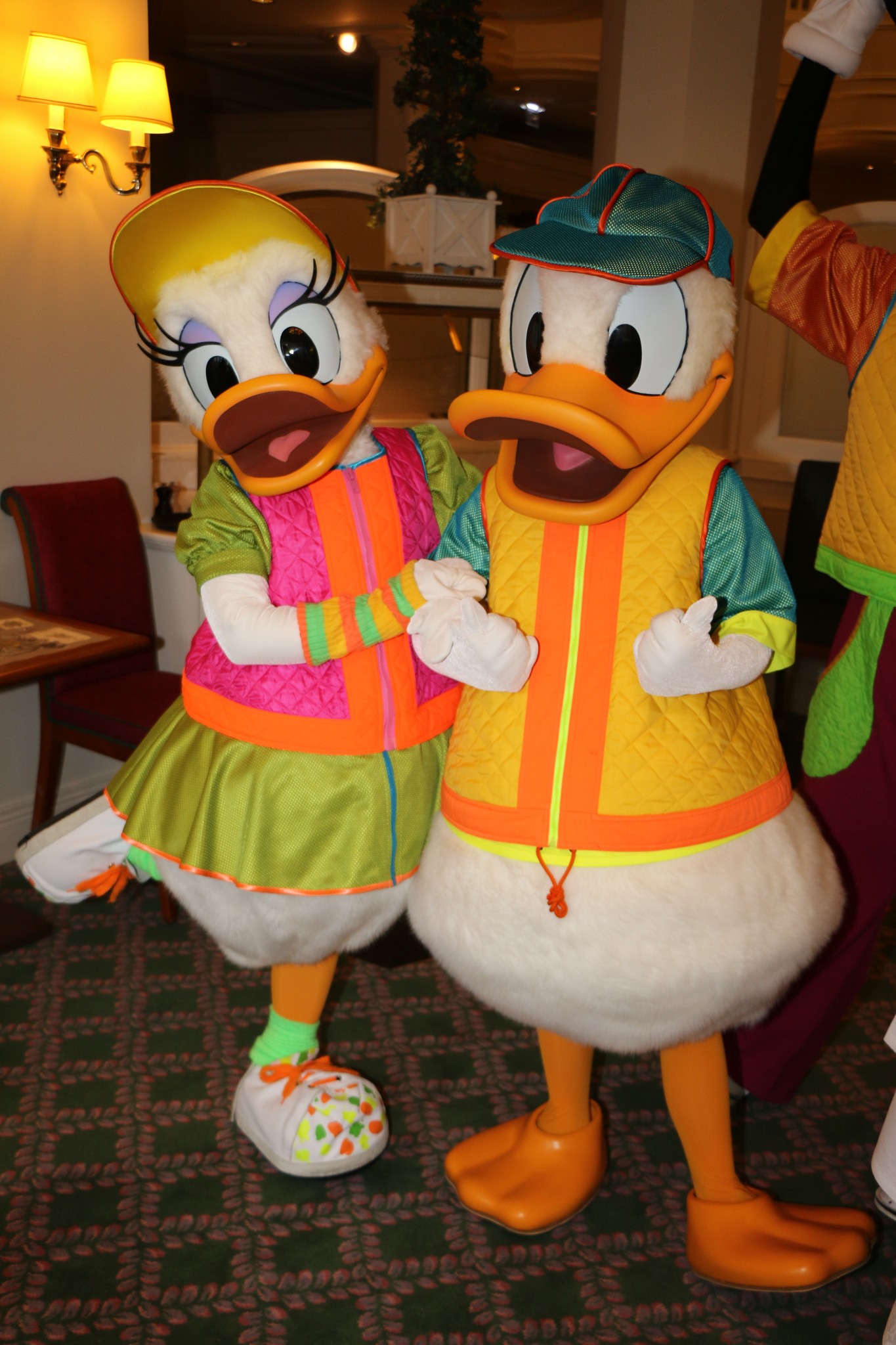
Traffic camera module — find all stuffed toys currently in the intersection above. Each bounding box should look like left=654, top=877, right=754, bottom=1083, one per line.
left=725, top=0, right=896, bottom=1225
left=14, top=180, right=484, bottom=1177
left=403, top=163, right=873, bottom=1293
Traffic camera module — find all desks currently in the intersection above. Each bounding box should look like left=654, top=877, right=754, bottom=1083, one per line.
left=0, top=600, right=165, bottom=691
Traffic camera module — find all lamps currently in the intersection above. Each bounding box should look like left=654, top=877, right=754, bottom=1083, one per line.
left=15, top=32, right=174, bottom=199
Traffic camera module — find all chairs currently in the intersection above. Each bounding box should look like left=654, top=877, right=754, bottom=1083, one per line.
left=0, top=476, right=180, bottom=834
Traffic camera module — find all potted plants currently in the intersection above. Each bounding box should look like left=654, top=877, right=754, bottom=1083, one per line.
left=370, top=0, right=501, bottom=275
